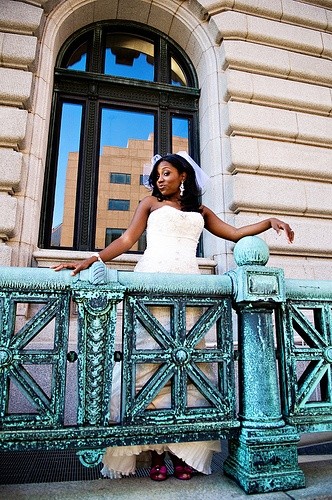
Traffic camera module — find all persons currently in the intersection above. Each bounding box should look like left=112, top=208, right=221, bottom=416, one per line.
left=50, top=151, right=295, bottom=480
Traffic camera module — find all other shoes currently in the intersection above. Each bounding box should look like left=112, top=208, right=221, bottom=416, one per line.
left=174, top=464, right=192, bottom=479
left=150, top=462, right=167, bottom=480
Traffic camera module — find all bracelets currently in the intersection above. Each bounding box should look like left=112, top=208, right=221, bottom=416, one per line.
left=89, top=253, right=106, bottom=266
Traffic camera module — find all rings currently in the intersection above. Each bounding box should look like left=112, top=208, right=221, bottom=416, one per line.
left=290, top=229, right=293, bottom=232
